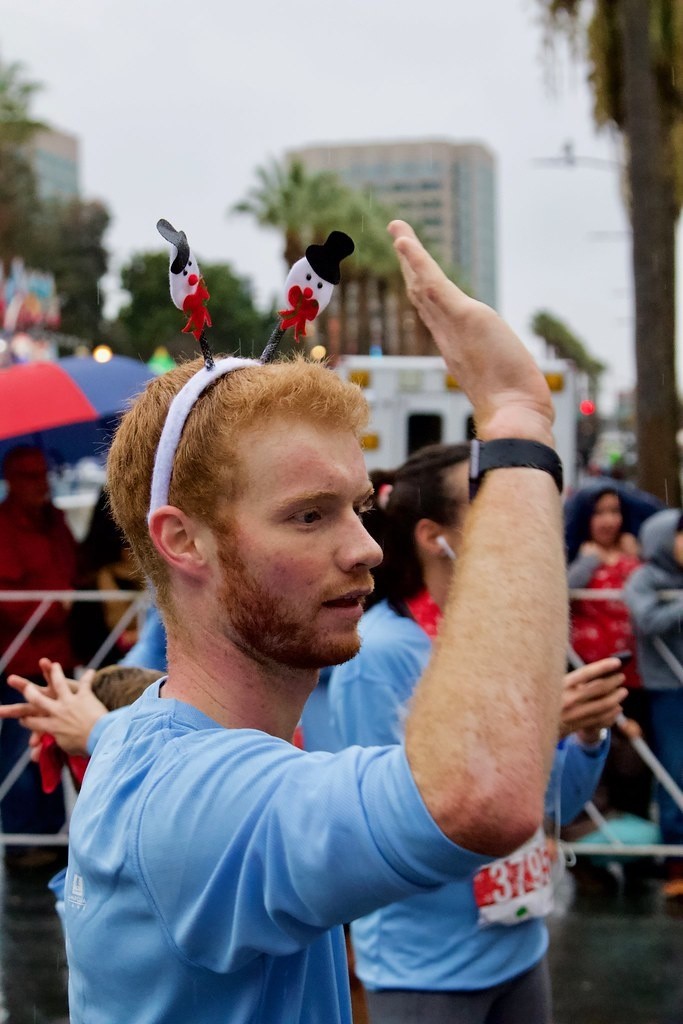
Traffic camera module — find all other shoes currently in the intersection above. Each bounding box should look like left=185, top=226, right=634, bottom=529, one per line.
left=566, top=855, right=619, bottom=898
left=619, top=855, right=659, bottom=893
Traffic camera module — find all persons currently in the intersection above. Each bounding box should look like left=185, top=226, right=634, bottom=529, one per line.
left=546, top=481, right=683, bottom=903
left=0, top=444, right=81, bottom=918
left=63, top=220, right=571, bottom=1024
left=333, top=442, right=627, bottom=1024
left=0, top=658, right=167, bottom=761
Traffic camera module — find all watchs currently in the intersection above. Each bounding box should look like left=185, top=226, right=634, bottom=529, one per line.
left=467, top=438, right=564, bottom=500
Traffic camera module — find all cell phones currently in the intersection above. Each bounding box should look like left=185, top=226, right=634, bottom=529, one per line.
left=583, top=648, right=635, bottom=687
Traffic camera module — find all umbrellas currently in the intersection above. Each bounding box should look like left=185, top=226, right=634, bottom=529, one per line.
left=0, top=353, right=163, bottom=475
